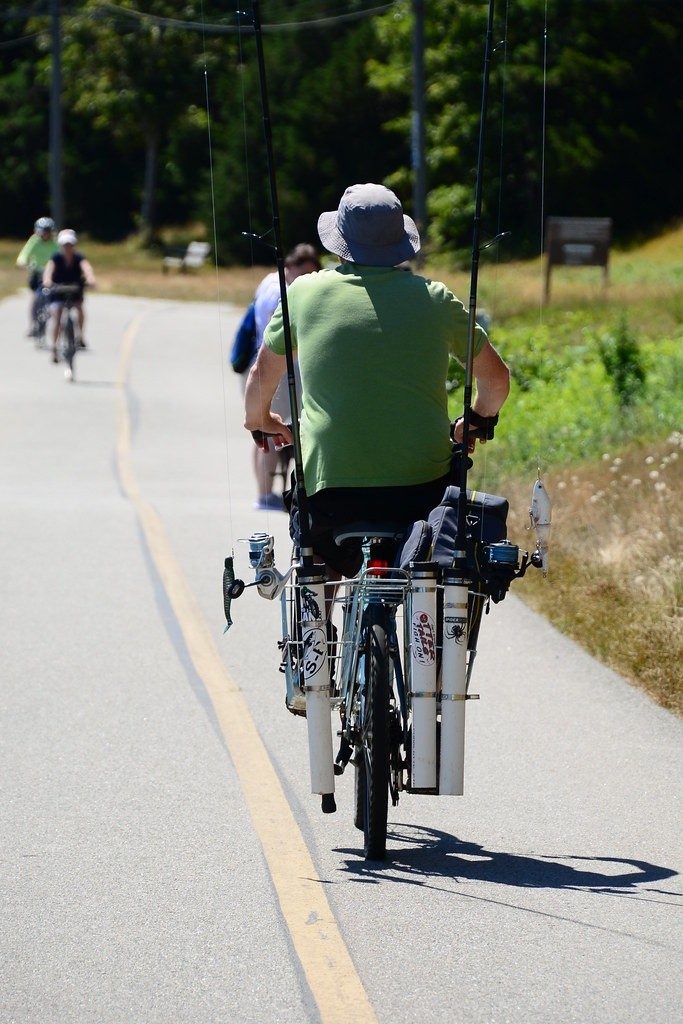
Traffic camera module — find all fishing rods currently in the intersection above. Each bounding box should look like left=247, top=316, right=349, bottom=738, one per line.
left=453, top=0, right=553, bottom=579
left=220, top=0, right=329, bottom=635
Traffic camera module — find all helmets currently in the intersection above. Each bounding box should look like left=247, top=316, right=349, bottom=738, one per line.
left=35, top=218, right=55, bottom=229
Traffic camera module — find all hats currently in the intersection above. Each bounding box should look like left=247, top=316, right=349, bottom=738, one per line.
left=58, top=229, right=78, bottom=245
left=318, top=183, right=421, bottom=265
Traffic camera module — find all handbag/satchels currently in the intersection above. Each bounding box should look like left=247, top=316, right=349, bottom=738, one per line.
left=231, top=299, right=260, bottom=374
left=400, top=486, right=509, bottom=604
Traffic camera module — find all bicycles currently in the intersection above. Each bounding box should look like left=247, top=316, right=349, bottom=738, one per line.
left=26, top=260, right=88, bottom=381
left=259, top=421, right=494, bottom=862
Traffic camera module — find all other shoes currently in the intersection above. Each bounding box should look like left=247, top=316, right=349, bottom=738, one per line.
left=258, top=494, right=284, bottom=511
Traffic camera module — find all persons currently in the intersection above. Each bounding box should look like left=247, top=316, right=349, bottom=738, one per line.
left=241, top=244, right=322, bottom=509
left=243, top=184, right=511, bottom=713
left=43, top=230, right=96, bottom=363
left=16, top=218, right=59, bottom=338
